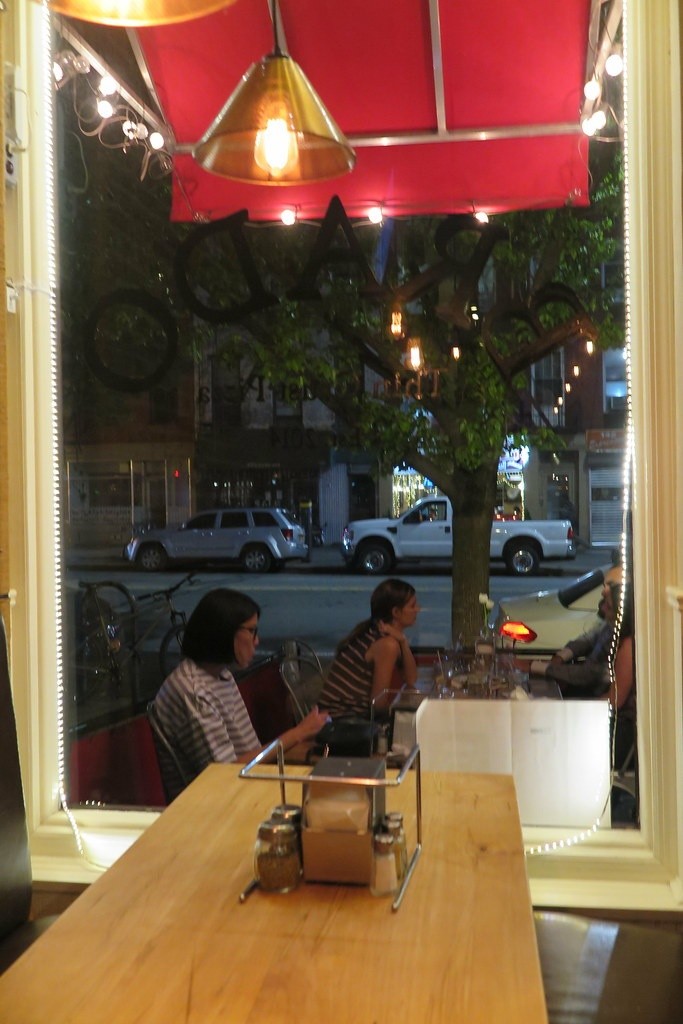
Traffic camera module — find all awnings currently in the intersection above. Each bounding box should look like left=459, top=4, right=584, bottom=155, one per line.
left=114, top=1, right=592, bottom=224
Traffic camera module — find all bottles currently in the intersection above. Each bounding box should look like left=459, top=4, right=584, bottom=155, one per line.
left=254, top=803, right=303, bottom=893
left=370, top=812, right=406, bottom=898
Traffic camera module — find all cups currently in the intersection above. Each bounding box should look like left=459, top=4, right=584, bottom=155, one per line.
left=437, top=638, right=529, bottom=699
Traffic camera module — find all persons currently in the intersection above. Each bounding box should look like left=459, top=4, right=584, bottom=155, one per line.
left=148, top=586, right=330, bottom=806
left=316, top=580, right=420, bottom=756
left=511, top=567, right=639, bottom=773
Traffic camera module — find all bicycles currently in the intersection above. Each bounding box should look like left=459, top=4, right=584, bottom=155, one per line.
left=74, top=570, right=197, bottom=705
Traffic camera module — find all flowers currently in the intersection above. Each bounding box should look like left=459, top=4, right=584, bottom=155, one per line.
left=480, top=593, right=496, bottom=627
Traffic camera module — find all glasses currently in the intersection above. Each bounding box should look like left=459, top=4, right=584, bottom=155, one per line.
left=239, top=624, right=259, bottom=639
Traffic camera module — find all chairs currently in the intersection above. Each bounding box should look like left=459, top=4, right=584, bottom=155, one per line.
left=147, top=699, right=186, bottom=807
left=275, top=637, right=325, bottom=726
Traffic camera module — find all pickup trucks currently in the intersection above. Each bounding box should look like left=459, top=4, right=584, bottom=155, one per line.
left=341, top=496, right=576, bottom=576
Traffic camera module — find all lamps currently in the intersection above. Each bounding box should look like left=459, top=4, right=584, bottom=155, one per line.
left=194, top=1, right=356, bottom=182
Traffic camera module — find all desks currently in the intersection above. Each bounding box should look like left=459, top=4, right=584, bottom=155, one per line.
left=388, top=664, right=563, bottom=752
left=1, top=762, right=547, bottom=1024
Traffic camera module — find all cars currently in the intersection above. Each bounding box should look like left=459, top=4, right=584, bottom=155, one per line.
left=124, top=508, right=308, bottom=572
left=494, top=564, right=616, bottom=661
